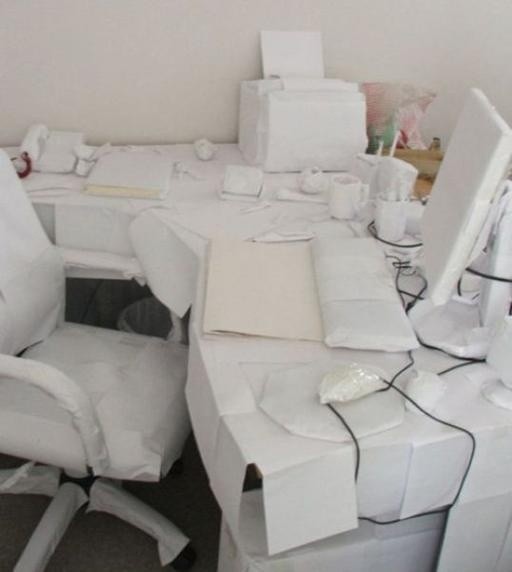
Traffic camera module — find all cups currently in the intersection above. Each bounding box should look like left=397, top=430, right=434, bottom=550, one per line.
left=374, top=194, right=409, bottom=242
left=329, top=174, right=371, bottom=220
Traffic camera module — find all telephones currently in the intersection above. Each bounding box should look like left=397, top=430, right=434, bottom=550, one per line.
left=13, top=124, right=84, bottom=173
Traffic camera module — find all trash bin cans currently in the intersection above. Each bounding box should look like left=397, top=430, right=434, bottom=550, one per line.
left=118, top=296, right=190, bottom=344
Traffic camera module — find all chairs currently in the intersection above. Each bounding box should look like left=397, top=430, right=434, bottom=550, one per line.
left=1, top=146, right=188, bottom=571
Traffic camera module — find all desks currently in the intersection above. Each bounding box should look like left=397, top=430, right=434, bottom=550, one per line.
left=1, top=144, right=512, bottom=571
